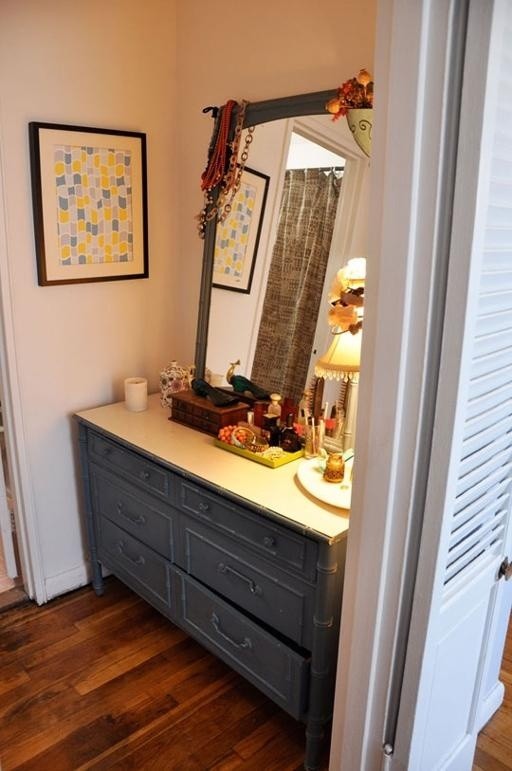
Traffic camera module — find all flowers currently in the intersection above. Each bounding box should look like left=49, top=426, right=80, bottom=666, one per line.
left=325, top=66, right=374, bottom=120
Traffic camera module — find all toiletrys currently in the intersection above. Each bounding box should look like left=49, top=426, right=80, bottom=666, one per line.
left=247, top=393, right=302, bottom=455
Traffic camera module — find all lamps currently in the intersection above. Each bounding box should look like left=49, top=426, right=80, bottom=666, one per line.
left=328, top=257, right=366, bottom=308
left=297, top=307, right=366, bottom=511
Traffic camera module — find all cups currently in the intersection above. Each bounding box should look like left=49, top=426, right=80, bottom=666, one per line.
left=299, top=419, right=326, bottom=458
left=123, top=376, right=148, bottom=413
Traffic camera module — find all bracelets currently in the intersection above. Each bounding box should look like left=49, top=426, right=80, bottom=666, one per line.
left=217, top=425, right=270, bottom=456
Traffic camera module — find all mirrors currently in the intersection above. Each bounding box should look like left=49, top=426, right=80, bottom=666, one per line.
left=193, top=86, right=370, bottom=453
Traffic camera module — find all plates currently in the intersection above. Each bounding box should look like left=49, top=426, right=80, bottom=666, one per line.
left=295, top=456, right=354, bottom=511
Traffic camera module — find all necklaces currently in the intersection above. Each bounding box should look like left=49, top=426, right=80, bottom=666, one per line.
left=200, top=98, right=238, bottom=192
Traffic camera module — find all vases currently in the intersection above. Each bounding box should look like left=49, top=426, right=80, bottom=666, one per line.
left=345, top=108, right=373, bottom=157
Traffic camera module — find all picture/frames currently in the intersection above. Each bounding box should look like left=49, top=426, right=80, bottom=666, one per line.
left=29, top=121, right=149, bottom=286
left=212, top=161, right=270, bottom=294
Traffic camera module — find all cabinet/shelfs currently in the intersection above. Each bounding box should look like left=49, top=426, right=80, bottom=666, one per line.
left=72, top=391, right=351, bottom=771
